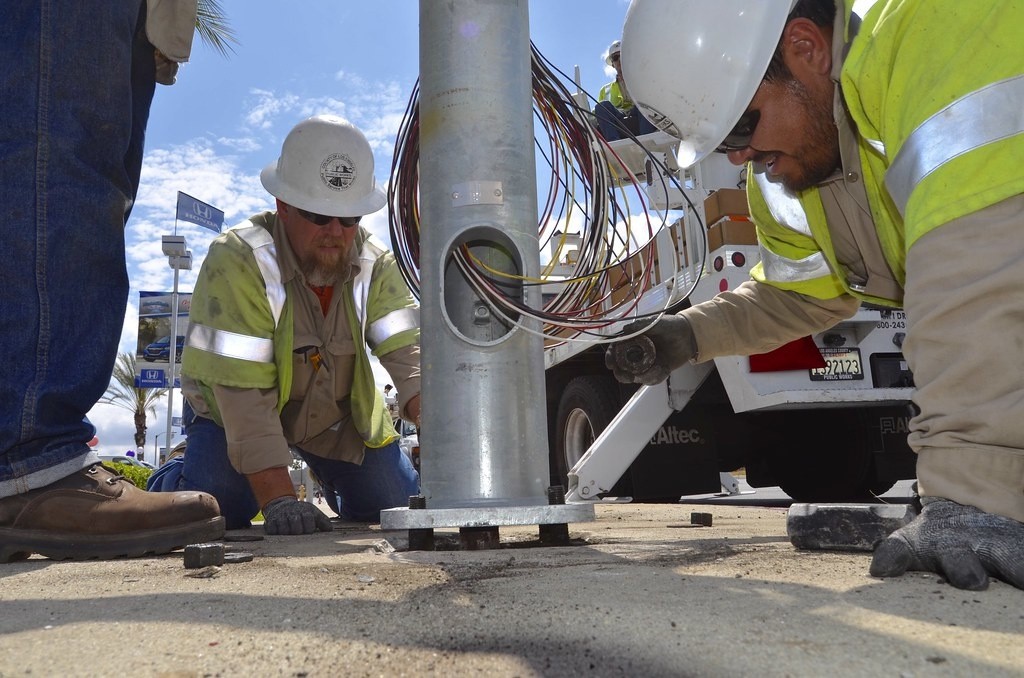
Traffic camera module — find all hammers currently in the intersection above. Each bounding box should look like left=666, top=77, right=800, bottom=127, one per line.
left=785, top=502, right=916, bottom=552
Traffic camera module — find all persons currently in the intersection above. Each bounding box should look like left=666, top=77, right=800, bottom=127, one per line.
left=594, top=40, right=655, bottom=142
left=604, top=0, right=1024, bottom=591
left=0, top=1, right=227, bottom=564
left=144, top=114, right=421, bottom=535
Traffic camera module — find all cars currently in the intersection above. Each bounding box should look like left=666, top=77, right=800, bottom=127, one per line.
left=143, top=335, right=186, bottom=363
left=399, top=416, right=419, bottom=470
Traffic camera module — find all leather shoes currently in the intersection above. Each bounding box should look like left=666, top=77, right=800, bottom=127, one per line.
left=0, top=462, right=226, bottom=560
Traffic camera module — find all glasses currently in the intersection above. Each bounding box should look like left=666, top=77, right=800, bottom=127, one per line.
left=610, top=54, right=620, bottom=63
left=296, top=208, right=362, bottom=228
left=713, top=107, right=763, bottom=154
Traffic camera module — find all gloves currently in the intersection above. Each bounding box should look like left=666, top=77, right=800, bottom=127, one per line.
left=262, top=495, right=334, bottom=535
left=869, top=497, right=1024, bottom=589
left=604, top=309, right=694, bottom=384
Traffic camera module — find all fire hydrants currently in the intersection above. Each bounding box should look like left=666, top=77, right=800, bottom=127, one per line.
left=298, top=484, right=306, bottom=501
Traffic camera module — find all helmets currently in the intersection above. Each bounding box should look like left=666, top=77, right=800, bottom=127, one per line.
left=605, top=41, right=620, bottom=66
left=259, top=114, right=388, bottom=217
left=620, top=1, right=794, bottom=169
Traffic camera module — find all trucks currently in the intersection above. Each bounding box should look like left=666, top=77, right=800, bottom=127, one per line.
left=97, top=455, right=160, bottom=470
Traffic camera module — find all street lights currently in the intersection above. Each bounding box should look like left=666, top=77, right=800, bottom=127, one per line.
left=154, top=432, right=177, bottom=466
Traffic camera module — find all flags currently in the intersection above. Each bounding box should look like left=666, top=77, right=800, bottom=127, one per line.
left=176, top=191, right=224, bottom=234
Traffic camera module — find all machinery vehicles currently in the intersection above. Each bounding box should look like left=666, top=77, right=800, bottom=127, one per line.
left=542, top=64, right=917, bottom=522
